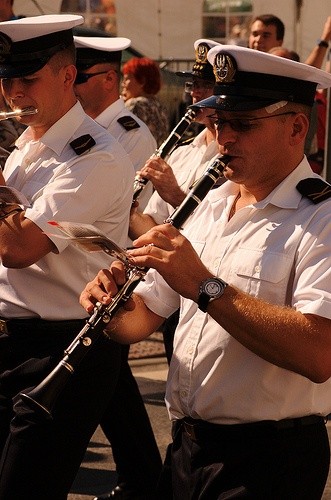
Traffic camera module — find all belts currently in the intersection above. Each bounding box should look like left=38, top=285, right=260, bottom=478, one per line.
left=183, top=415, right=319, bottom=442
left=0, top=319, right=106, bottom=338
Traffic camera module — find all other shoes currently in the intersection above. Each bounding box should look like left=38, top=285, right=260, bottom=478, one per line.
left=98, top=484, right=132, bottom=500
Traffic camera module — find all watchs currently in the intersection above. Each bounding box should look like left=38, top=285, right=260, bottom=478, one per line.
left=197, top=276, right=229, bottom=313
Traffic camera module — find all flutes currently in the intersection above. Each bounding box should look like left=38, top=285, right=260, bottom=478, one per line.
left=131, top=102, right=207, bottom=209
left=17, top=152, right=233, bottom=422
left=0, top=105, right=38, bottom=121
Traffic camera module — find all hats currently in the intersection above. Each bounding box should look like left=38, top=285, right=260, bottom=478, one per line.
left=176, top=39, right=223, bottom=81
left=187, top=45, right=331, bottom=111
left=73, top=35, right=131, bottom=71
left=0, top=15, right=84, bottom=78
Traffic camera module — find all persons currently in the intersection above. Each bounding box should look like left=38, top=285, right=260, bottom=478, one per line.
left=0, top=15, right=331, bottom=500
left=121, top=56, right=170, bottom=152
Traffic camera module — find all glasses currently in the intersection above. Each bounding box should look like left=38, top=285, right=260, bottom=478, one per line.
left=185, top=82, right=215, bottom=93
left=205, top=112, right=296, bottom=132
left=75, top=71, right=123, bottom=84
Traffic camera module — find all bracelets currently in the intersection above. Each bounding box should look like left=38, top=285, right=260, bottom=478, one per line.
left=317, top=40, right=328, bottom=47
